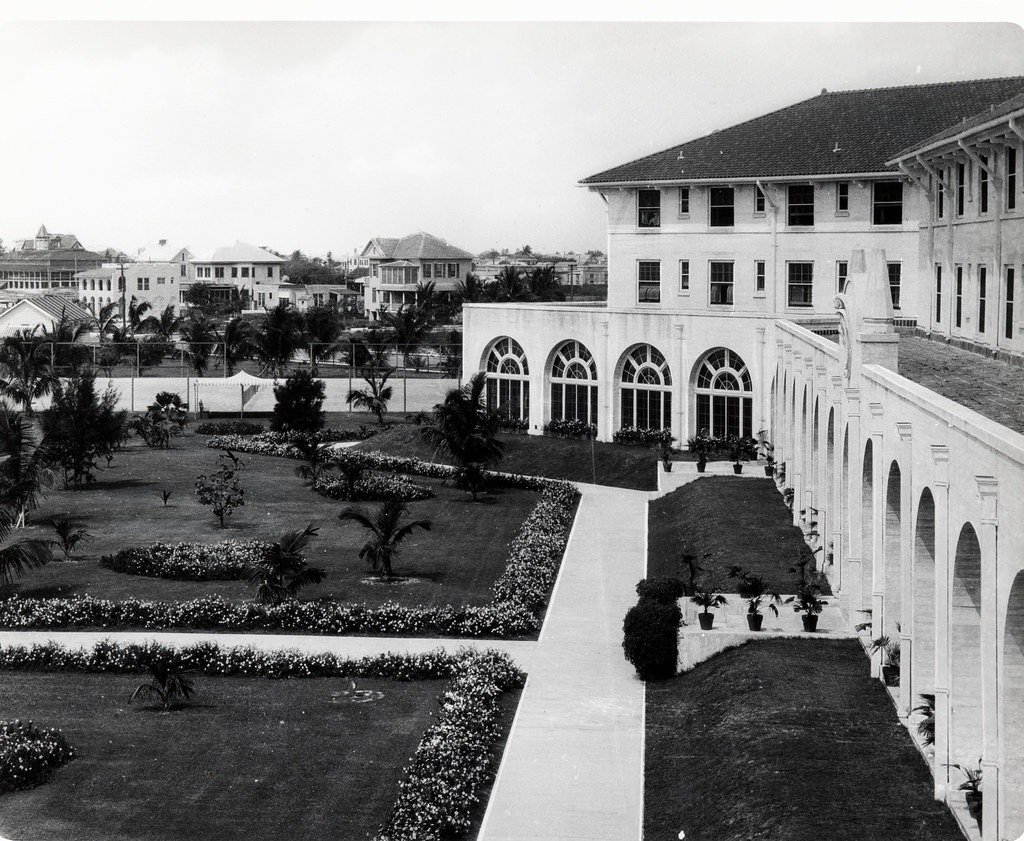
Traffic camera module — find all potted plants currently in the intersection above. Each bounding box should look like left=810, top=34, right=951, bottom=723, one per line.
left=680, top=553, right=712, bottom=596
left=869, top=623, right=901, bottom=688
left=759, top=441, right=773, bottom=476
left=911, top=694, right=935, bottom=748
left=944, top=761, right=982, bottom=833
left=659, top=433, right=676, bottom=472
left=688, top=587, right=727, bottom=630
left=686, top=438, right=719, bottom=472
left=724, top=439, right=759, bottom=474
left=783, top=580, right=829, bottom=632
left=727, top=566, right=782, bottom=631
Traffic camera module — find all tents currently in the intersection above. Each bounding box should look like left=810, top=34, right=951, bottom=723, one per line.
left=194, top=370, right=275, bottom=420
